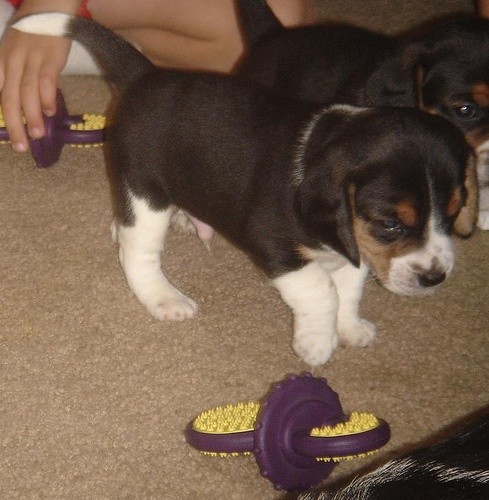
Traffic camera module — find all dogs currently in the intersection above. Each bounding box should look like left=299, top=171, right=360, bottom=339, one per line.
left=225, top=0, right=489, bottom=233
left=9, top=11, right=480, bottom=369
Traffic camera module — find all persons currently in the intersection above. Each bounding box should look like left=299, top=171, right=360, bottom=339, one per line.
left=0, top=0, right=315, bottom=152
